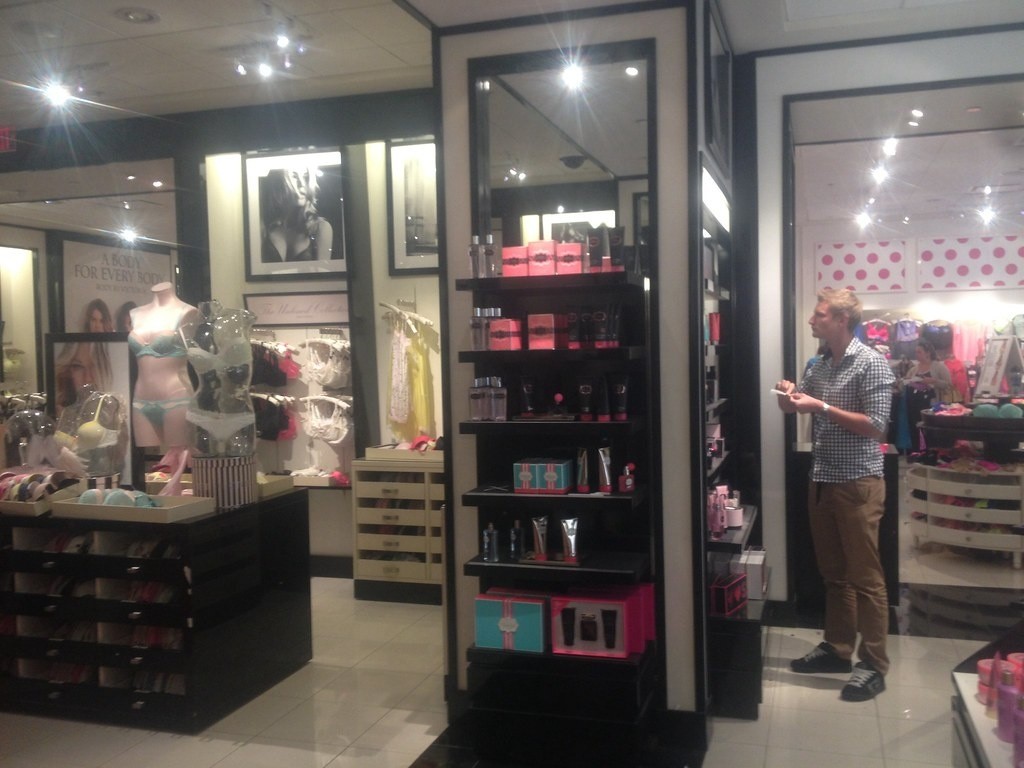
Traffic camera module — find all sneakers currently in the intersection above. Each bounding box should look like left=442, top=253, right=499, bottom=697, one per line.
left=842, top=662, right=885, bottom=701
left=791, top=642, right=852, bottom=673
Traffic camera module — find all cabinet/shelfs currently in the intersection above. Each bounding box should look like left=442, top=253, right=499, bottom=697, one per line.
left=702, top=277, right=773, bottom=722
left=456, top=260, right=659, bottom=739
left=788, top=441, right=899, bottom=610
left=0, top=486, right=314, bottom=736
left=351, top=456, right=444, bottom=606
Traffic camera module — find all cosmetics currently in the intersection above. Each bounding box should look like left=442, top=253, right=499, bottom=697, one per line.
left=505, top=443, right=639, bottom=495
left=700, top=278, right=746, bottom=536
left=467, top=304, right=622, bottom=353
left=466, top=368, right=630, bottom=425
left=977, top=649, right=1024, bottom=768
left=560, top=605, right=619, bottom=650
left=463, top=226, right=628, bottom=284
left=474, top=511, right=580, bottom=563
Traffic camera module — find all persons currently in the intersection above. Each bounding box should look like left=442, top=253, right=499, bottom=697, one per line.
left=126, top=281, right=201, bottom=446
left=178, top=299, right=258, bottom=459
left=904, top=340, right=953, bottom=408
left=261, top=165, right=335, bottom=264
left=50, top=298, right=138, bottom=410
left=773, top=290, right=892, bottom=701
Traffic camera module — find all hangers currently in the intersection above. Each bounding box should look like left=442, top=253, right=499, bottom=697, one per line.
left=378, top=298, right=434, bottom=335
left=299, top=385, right=354, bottom=415
left=249, top=329, right=296, bottom=358
left=248, top=385, right=295, bottom=408
left=299, top=328, right=351, bottom=359
left=858, top=311, right=951, bottom=328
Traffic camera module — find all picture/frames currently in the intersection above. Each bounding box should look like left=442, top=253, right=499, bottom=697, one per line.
left=241, top=146, right=347, bottom=283
left=384, top=139, right=439, bottom=275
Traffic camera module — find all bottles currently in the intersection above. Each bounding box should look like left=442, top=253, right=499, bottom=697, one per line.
left=997, top=671, right=1020, bottom=743
left=1014, top=694, right=1024, bottom=768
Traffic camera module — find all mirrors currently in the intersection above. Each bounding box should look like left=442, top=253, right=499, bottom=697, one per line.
left=467, top=38, right=659, bottom=272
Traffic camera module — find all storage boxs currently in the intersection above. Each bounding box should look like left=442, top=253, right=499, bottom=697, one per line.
left=567, top=585, right=647, bottom=654
left=555, top=242, right=581, bottom=274
left=474, top=594, right=546, bottom=653
left=610, top=582, right=656, bottom=641
left=527, top=241, right=556, bottom=276
left=0, top=478, right=85, bottom=517
left=51, top=496, right=218, bottom=524
left=729, top=550, right=768, bottom=601
left=528, top=313, right=566, bottom=350
left=502, top=246, right=528, bottom=277
left=536, top=457, right=575, bottom=495
left=487, top=585, right=551, bottom=645
left=488, top=318, right=523, bottom=351
left=512, top=457, right=544, bottom=494
left=365, top=443, right=444, bottom=463
left=146, top=471, right=293, bottom=499
left=550, top=589, right=634, bottom=659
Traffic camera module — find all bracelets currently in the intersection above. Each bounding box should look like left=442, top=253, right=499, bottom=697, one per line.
left=822, top=404, right=830, bottom=418
